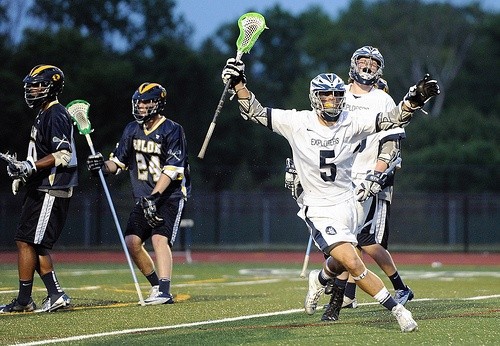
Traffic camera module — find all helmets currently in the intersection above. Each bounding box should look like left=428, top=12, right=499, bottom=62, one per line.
left=309, top=73, right=346, bottom=122
left=349, top=45, right=384, bottom=86
left=131, top=82, right=166, bottom=125
left=373, top=77, right=389, bottom=93
left=22, top=64, right=65, bottom=110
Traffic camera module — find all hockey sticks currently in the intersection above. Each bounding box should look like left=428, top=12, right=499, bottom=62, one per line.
left=355, top=157, right=403, bottom=201
left=197, top=12, right=267, bottom=159
left=0, top=152, right=26, bottom=185
left=65, top=99, right=147, bottom=307
left=299, top=235, right=312, bottom=279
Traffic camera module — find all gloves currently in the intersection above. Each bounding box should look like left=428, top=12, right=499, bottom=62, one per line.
left=86, top=153, right=104, bottom=171
left=6, top=159, right=37, bottom=177
left=355, top=170, right=387, bottom=203
left=140, top=197, right=164, bottom=229
left=221, top=59, right=247, bottom=88
left=12, top=172, right=30, bottom=195
left=404, top=74, right=440, bottom=110
left=285, top=159, right=298, bottom=189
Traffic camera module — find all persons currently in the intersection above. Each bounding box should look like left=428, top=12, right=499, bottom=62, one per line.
left=284, top=45, right=414, bottom=320
left=0, top=63, right=77, bottom=313
left=84, top=82, right=190, bottom=307
left=220, top=52, right=440, bottom=331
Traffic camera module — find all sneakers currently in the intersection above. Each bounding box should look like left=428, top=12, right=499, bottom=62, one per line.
left=33, top=292, right=71, bottom=314
left=392, top=306, right=417, bottom=332
left=305, top=270, right=326, bottom=315
left=392, top=285, right=414, bottom=305
left=0, top=298, right=36, bottom=314
left=323, top=295, right=358, bottom=310
left=138, top=292, right=175, bottom=306
left=321, top=284, right=346, bottom=321
left=148, top=286, right=158, bottom=298
